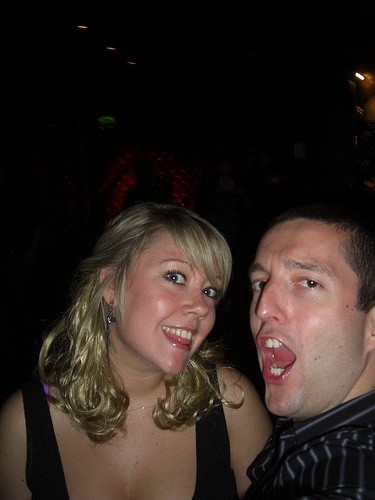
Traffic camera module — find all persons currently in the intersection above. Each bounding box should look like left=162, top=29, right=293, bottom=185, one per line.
left=0, top=205, right=274, bottom=500
left=210, top=159, right=375, bottom=500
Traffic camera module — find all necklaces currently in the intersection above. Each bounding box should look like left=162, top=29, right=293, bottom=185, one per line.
left=221, top=176, right=234, bottom=190
